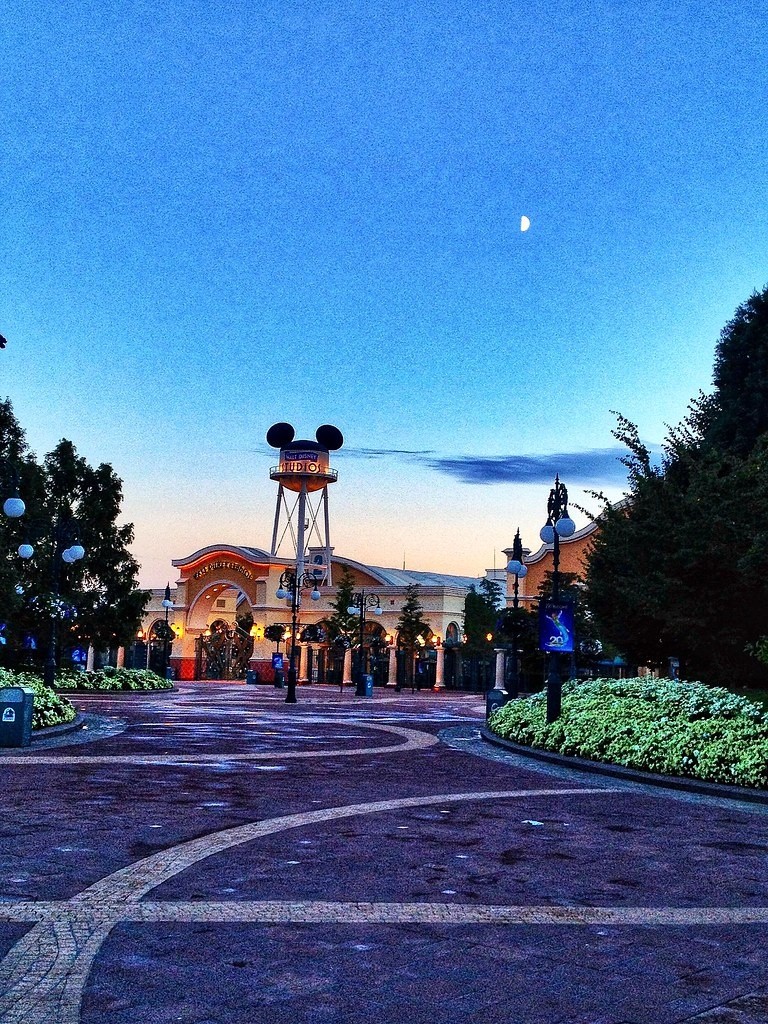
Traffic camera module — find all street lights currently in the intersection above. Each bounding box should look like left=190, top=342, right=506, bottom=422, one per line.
left=536, top=471, right=576, bottom=724
left=42, top=510, right=86, bottom=688
left=274, top=570, right=323, bottom=702
left=345, top=588, right=385, bottom=673
left=161, top=582, right=177, bottom=682
left=506, top=528, right=535, bottom=698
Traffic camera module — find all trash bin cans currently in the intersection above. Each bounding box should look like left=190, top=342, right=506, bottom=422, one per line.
left=166, top=666, right=176, bottom=680
left=276, top=671, right=284, bottom=688
left=0, top=685, right=35, bottom=748
left=486, top=689, right=508, bottom=721
left=247, top=670, right=258, bottom=684
left=360, top=673, right=374, bottom=697
